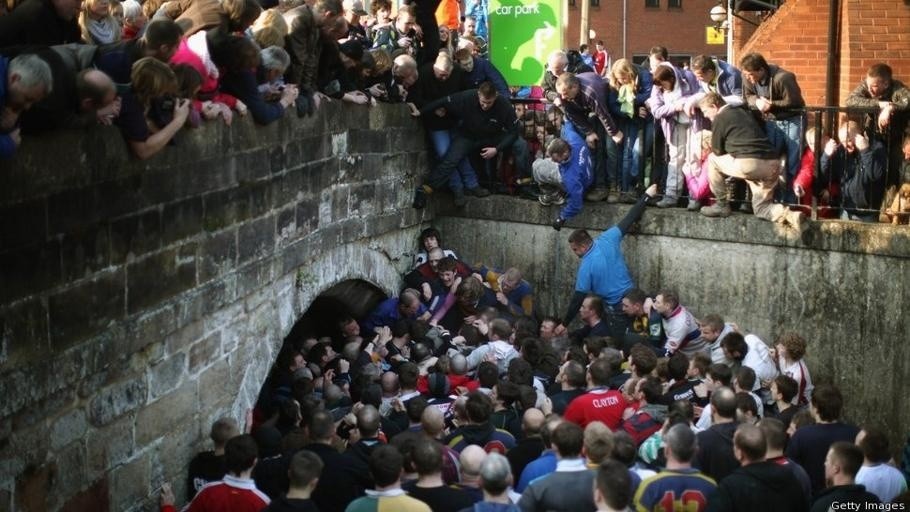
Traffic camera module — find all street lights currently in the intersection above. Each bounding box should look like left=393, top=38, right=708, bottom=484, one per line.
left=712, top=6, right=733, bottom=66
left=589, top=29, right=597, bottom=48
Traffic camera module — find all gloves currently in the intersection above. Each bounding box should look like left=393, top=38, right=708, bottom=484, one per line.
left=553, top=217, right=567, bottom=230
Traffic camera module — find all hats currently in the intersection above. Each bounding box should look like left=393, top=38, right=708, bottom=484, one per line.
left=339, top=40, right=363, bottom=63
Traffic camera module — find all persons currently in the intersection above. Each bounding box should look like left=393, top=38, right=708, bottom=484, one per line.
left=1, top=1, right=910, bottom=511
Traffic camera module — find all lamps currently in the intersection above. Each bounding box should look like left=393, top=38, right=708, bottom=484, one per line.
left=710, top=2, right=730, bottom=32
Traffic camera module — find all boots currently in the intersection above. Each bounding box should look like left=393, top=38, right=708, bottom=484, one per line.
left=699, top=201, right=731, bottom=216
left=780, top=209, right=815, bottom=245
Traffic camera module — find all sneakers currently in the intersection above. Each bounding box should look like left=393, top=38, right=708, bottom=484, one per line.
left=586, top=189, right=641, bottom=203
left=471, top=188, right=490, bottom=197
left=656, top=198, right=678, bottom=208
left=519, top=188, right=540, bottom=200
left=453, top=190, right=465, bottom=206
left=538, top=192, right=565, bottom=206
left=686, top=201, right=701, bottom=210
left=415, top=188, right=428, bottom=208
